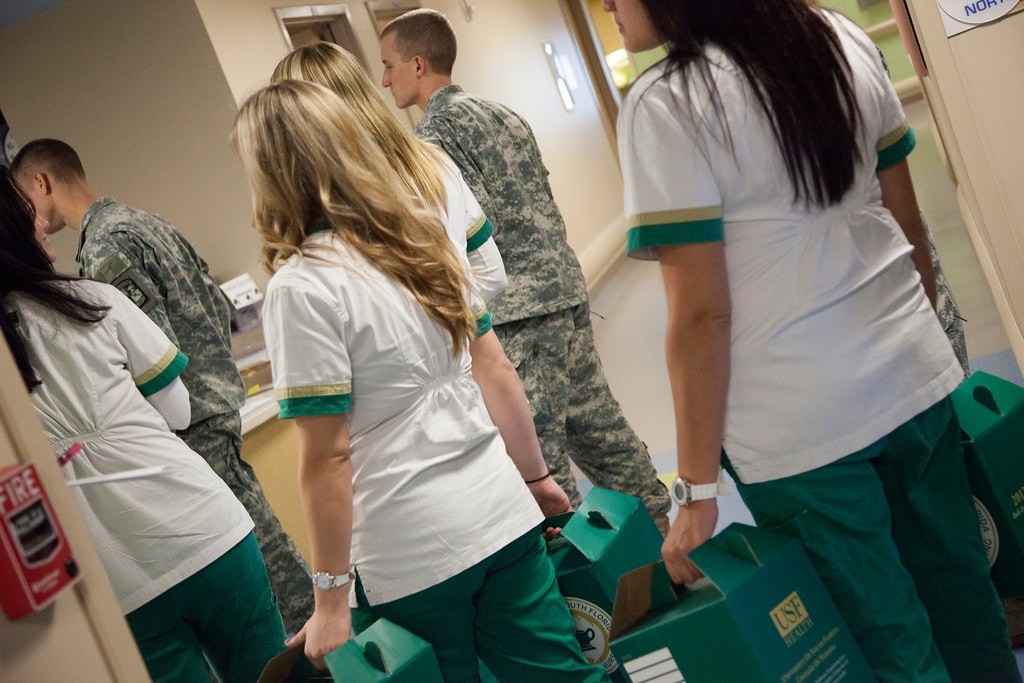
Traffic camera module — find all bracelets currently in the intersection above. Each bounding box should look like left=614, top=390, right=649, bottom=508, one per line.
left=526, top=471, right=555, bottom=482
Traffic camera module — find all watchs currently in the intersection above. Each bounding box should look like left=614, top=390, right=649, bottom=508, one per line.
left=673, top=475, right=722, bottom=507
left=310, top=571, right=357, bottom=590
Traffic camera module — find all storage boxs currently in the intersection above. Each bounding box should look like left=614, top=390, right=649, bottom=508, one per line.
left=253, top=618, right=443, bottom=683
left=609, top=523, right=875, bottom=683
left=951, top=372, right=1024, bottom=602
left=544, top=486, right=680, bottom=683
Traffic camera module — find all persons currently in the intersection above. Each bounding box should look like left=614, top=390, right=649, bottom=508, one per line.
left=0, top=165, right=290, bottom=683
left=603, top=0, right=1024, bottom=683
left=229, top=80, right=614, bottom=683
left=9, top=138, right=315, bottom=634
left=378, top=9, right=671, bottom=538
left=268, top=42, right=506, bottom=304
